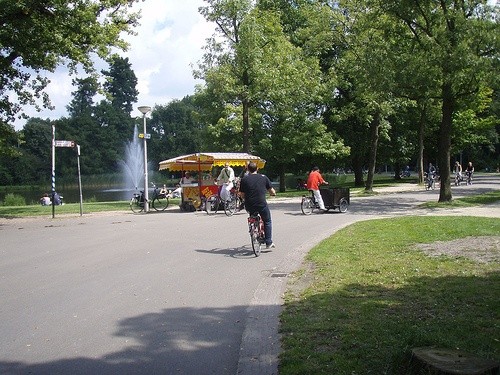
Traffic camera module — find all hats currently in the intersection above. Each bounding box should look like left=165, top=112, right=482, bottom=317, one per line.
left=185, top=171, right=190, bottom=175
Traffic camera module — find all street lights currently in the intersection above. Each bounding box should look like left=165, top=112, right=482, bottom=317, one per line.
left=138, top=105, right=152, bottom=213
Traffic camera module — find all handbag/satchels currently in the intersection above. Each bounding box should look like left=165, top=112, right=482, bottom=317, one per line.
left=232, top=180, right=237, bottom=188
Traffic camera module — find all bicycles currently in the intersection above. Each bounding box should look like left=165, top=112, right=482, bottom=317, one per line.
left=130, top=182, right=169, bottom=213
left=424, top=171, right=435, bottom=191
left=205, top=181, right=245, bottom=217
left=454, top=172, right=462, bottom=186
left=239, top=194, right=276, bottom=257
left=465, top=171, right=473, bottom=186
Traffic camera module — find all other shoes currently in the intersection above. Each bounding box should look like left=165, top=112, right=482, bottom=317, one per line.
left=266, top=242, right=276, bottom=248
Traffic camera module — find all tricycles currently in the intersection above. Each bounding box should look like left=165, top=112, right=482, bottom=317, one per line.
left=300, top=183, right=350, bottom=215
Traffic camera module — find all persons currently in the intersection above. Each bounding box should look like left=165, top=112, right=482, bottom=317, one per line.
left=465, top=162, right=474, bottom=180
left=40, top=193, right=52, bottom=205
left=307, top=166, right=329, bottom=211
left=426, top=163, right=436, bottom=183
left=160, top=184, right=171, bottom=198
left=236, top=161, right=252, bottom=200
left=172, top=184, right=183, bottom=198
left=296, top=178, right=305, bottom=190
left=53, top=192, right=62, bottom=205
left=179, top=171, right=195, bottom=185
left=217, top=162, right=234, bottom=202
left=238, top=162, right=276, bottom=248
left=454, top=161, right=462, bottom=179
